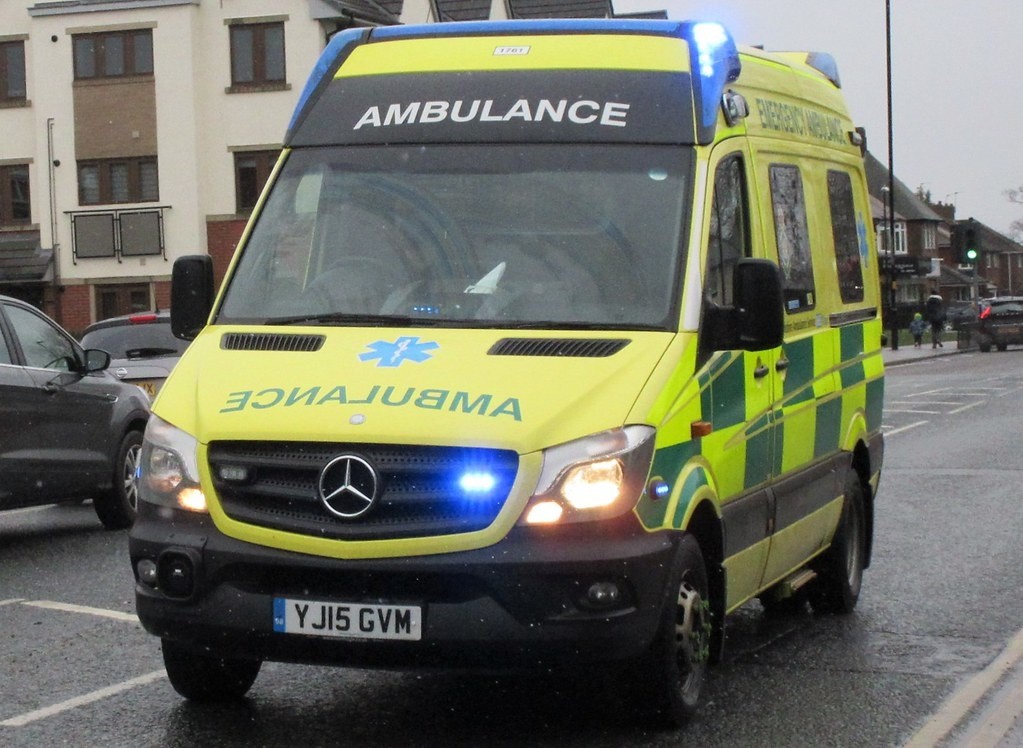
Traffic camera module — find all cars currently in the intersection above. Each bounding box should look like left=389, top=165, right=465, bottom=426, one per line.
left=78, top=310, right=193, bottom=404
left=977, top=296, right=1023, bottom=352
left=0, top=295, right=153, bottom=529
left=952, top=298, right=994, bottom=330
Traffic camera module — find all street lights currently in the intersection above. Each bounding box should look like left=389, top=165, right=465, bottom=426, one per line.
left=880, top=184, right=889, bottom=283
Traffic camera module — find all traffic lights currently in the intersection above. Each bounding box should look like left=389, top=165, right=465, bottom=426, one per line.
left=963, top=223, right=981, bottom=263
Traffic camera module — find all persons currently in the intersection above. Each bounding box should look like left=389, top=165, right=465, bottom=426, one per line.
left=909, top=313, right=930, bottom=348
left=927, top=289, right=944, bottom=348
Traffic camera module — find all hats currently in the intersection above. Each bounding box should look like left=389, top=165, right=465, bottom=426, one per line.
left=915, top=312, right=921, bottom=320
left=932, top=289, right=938, bottom=294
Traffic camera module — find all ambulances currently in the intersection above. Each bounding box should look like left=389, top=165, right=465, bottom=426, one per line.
left=130, top=18, right=884, bottom=728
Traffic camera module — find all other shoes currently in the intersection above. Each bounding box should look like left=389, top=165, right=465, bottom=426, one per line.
left=932, top=345, right=936, bottom=348
left=914, top=342, right=917, bottom=346
left=938, top=341, right=943, bottom=347
